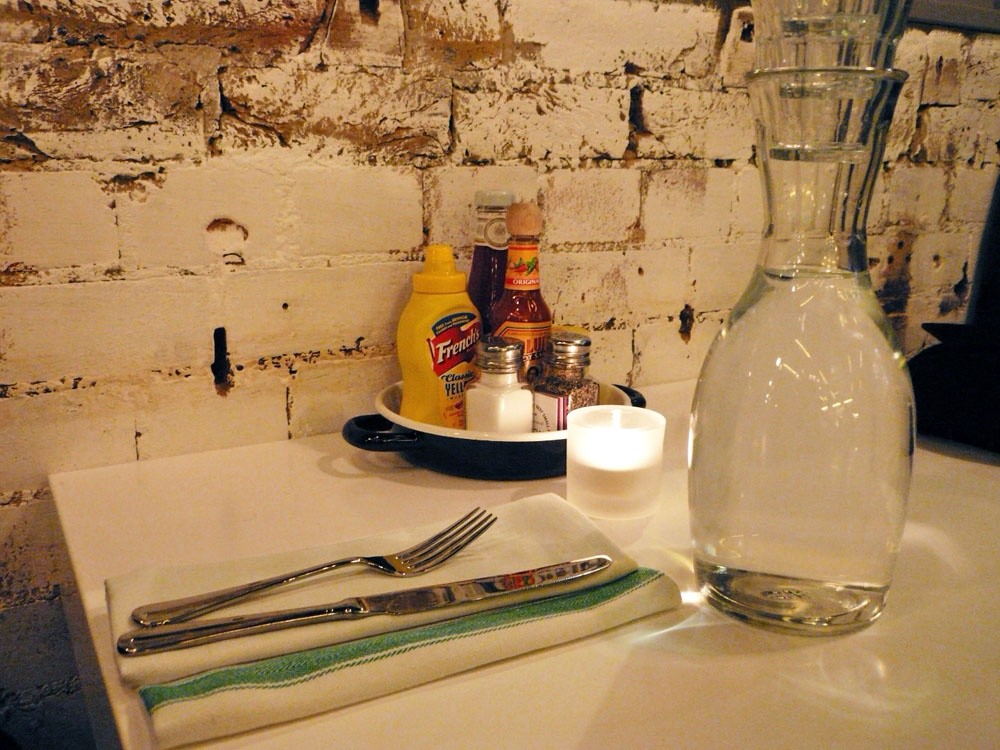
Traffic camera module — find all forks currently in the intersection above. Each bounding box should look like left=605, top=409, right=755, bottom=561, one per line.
left=131, top=507, right=498, bottom=627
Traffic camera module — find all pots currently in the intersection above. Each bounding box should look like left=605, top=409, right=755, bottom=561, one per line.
left=342, top=381, right=647, bottom=481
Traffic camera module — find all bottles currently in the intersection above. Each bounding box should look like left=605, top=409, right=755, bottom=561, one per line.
left=463, top=336, right=533, bottom=432
left=532, top=332, right=600, bottom=432
left=467, top=190, right=515, bottom=337
left=683, top=0, right=915, bottom=640
left=489, top=203, right=552, bottom=380
left=396, top=245, right=481, bottom=430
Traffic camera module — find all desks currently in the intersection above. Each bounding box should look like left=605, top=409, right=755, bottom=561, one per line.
left=48, top=379, right=1000, bottom=750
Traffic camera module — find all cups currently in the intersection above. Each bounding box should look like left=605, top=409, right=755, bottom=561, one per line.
left=565, top=404, right=665, bottom=519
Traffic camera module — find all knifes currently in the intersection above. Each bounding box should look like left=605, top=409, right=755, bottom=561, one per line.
left=117, top=554, right=610, bottom=657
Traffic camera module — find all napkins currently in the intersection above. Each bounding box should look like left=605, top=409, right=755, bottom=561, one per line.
left=101, top=499, right=680, bottom=750
